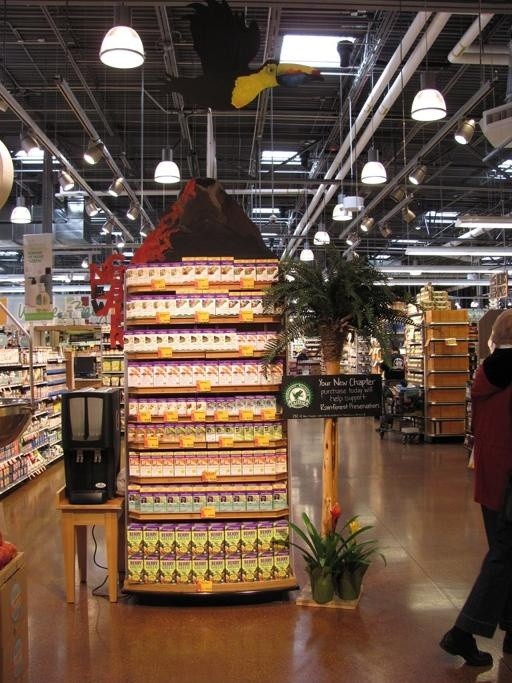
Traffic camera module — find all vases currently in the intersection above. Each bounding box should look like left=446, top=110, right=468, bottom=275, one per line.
left=335, top=562, right=364, bottom=603
left=306, top=565, right=334, bottom=604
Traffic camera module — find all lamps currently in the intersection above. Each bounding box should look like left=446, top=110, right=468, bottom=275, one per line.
left=332, top=161, right=353, bottom=222
left=360, top=218, right=375, bottom=232
left=108, top=174, right=125, bottom=198
left=140, top=222, right=151, bottom=238
left=122, top=247, right=136, bottom=258
left=57, top=170, right=74, bottom=191
left=154, top=95, right=180, bottom=184
left=361, top=68, right=387, bottom=184
left=99, top=2, right=146, bottom=69
left=346, top=232, right=359, bottom=246
left=379, top=223, right=392, bottom=239
left=389, top=184, right=406, bottom=202
left=347, top=251, right=359, bottom=262
left=409, top=164, right=428, bottom=186
left=81, top=258, right=89, bottom=267
left=20, top=127, right=40, bottom=157
left=83, top=196, right=98, bottom=217
left=102, top=217, right=115, bottom=234
left=127, top=201, right=141, bottom=221
left=401, top=207, right=417, bottom=225
left=454, top=120, right=479, bottom=145
left=115, top=232, right=127, bottom=249
left=411, top=11, right=447, bottom=121
left=314, top=192, right=330, bottom=246
left=84, top=137, right=104, bottom=165
left=10, top=171, right=31, bottom=224
left=300, top=225, right=314, bottom=262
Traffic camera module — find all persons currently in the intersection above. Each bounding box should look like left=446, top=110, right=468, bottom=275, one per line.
left=439, top=308, right=512, bottom=666
left=296, top=349, right=308, bottom=365
left=379, top=345, right=406, bottom=385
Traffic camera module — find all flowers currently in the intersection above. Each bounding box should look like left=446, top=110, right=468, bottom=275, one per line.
left=337, top=518, right=387, bottom=571
left=286, top=499, right=376, bottom=573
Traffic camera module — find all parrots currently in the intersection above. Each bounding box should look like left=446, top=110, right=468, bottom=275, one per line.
left=156, top=2, right=325, bottom=114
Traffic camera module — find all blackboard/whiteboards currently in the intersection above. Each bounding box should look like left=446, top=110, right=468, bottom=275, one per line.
left=281, top=374, right=383, bottom=419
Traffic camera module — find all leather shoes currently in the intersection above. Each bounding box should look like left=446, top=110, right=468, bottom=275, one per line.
left=439, top=629, right=493, bottom=668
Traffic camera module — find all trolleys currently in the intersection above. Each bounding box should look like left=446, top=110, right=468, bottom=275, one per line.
left=379, top=378, right=425, bottom=444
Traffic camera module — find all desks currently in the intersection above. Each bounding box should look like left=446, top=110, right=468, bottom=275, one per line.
left=54, top=482, right=123, bottom=605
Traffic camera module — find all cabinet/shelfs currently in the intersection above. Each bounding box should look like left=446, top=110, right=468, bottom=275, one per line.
left=284, top=272, right=512, bottom=444
left=0, top=537, right=31, bottom=682
left=0, top=287, right=127, bottom=498
left=118, top=262, right=295, bottom=593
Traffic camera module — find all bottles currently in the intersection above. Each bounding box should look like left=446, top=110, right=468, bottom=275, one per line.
left=40, top=265, right=53, bottom=304
left=0, top=344, right=48, bottom=401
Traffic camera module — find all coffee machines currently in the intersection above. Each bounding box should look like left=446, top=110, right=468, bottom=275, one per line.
left=60, top=386, right=123, bottom=505
